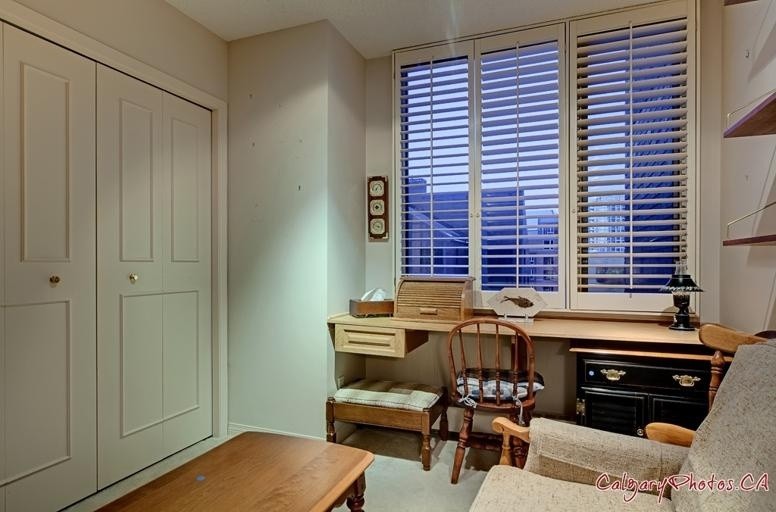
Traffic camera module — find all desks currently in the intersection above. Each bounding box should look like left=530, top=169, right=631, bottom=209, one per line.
left=329, top=313, right=701, bottom=345
left=89, top=429, right=375, bottom=512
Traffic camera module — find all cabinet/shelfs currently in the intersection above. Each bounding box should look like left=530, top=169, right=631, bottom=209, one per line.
left=1, top=20, right=213, bottom=510
left=570, top=347, right=732, bottom=448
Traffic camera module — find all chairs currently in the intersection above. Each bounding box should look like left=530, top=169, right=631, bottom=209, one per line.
left=448, top=318, right=776, bottom=511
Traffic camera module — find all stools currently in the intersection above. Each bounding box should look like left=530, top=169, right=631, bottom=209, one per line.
left=325, top=377, right=449, bottom=473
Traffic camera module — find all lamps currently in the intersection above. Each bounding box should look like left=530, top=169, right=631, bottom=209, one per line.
left=658, top=273, right=704, bottom=331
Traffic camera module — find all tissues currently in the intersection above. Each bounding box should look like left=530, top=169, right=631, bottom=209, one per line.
left=348, top=287, right=394, bottom=319
